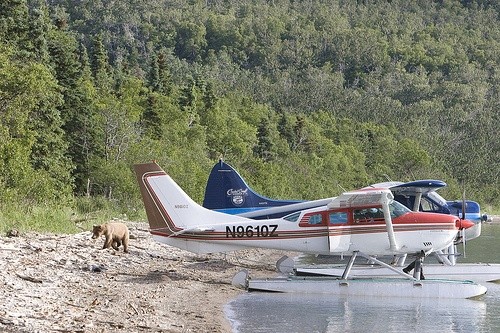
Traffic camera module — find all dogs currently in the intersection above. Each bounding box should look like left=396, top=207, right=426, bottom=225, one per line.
left=91, top=222, right=129, bottom=253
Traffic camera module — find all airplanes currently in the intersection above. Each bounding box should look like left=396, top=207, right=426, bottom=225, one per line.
left=203, top=158, right=493, bottom=270
left=133, top=159, right=470, bottom=288
